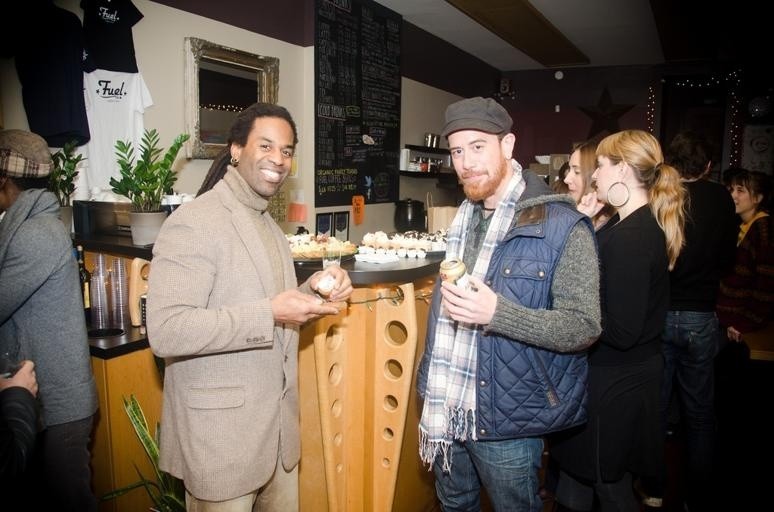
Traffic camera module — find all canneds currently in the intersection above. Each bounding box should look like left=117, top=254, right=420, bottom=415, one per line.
left=438, top=257, right=471, bottom=293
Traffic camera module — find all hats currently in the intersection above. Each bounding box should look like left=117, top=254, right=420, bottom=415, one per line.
left=440, top=97, right=514, bottom=139
left=0, top=129, right=55, bottom=181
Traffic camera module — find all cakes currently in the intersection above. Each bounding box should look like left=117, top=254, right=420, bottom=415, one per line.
left=281, top=231, right=358, bottom=259
left=315, top=275, right=336, bottom=296
left=354, top=228, right=446, bottom=264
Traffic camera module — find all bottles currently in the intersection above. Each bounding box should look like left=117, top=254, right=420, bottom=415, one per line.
left=410, top=156, right=443, bottom=173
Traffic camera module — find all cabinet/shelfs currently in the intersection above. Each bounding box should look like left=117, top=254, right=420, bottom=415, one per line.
left=400, top=145, right=454, bottom=179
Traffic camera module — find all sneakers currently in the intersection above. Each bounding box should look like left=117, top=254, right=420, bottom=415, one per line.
left=633, top=475, right=664, bottom=508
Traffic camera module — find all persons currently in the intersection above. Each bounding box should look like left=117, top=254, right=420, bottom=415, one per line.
left=145, top=102, right=356, bottom=512
left=1, top=358, right=42, bottom=512
left=1, top=128, right=104, bottom=511
left=556, top=129, right=774, bottom=512
left=413, top=95, right=603, bottom=510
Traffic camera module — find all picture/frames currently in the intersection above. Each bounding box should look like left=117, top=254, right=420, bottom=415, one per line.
left=184, top=37, right=279, bottom=161
left=315, top=211, right=350, bottom=243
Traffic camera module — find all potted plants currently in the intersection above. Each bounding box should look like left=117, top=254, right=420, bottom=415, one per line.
left=50, top=137, right=88, bottom=231
left=109, top=127, right=191, bottom=246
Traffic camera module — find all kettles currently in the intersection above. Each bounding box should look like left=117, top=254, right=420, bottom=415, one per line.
left=395, top=198, right=429, bottom=232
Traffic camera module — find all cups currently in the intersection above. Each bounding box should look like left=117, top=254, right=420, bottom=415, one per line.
left=160, top=191, right=196, bottom=205
left=400, top=149, right=410, bottom=171
left=425, top=132, right=440, bottom=147
left=1, top=350, right=25, bottom=378
left=89, top=254, right=129, bottom=330
left=75, top=245, right=90, bottom=325
left=322, top=249, right=341, bottom=268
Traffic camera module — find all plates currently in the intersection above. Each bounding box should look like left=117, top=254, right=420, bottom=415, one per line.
left=290, top=250, right=356, bottom=266
